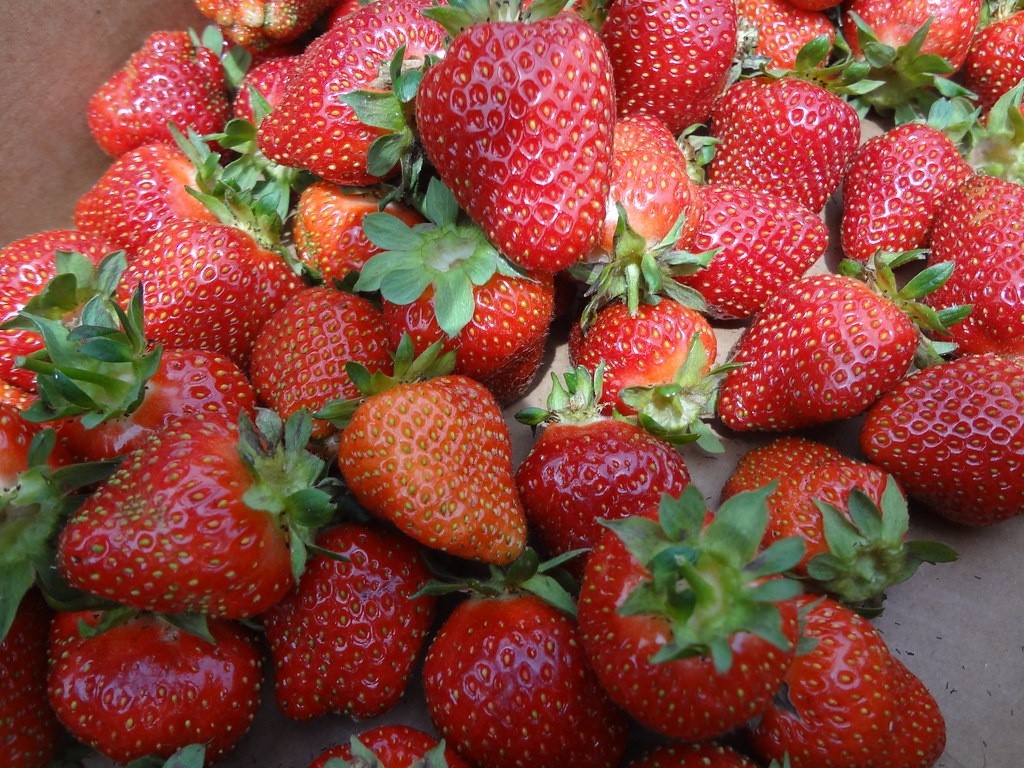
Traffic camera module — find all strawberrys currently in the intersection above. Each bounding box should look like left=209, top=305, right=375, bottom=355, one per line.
left=0, top=0, right=1024, bottom=768
